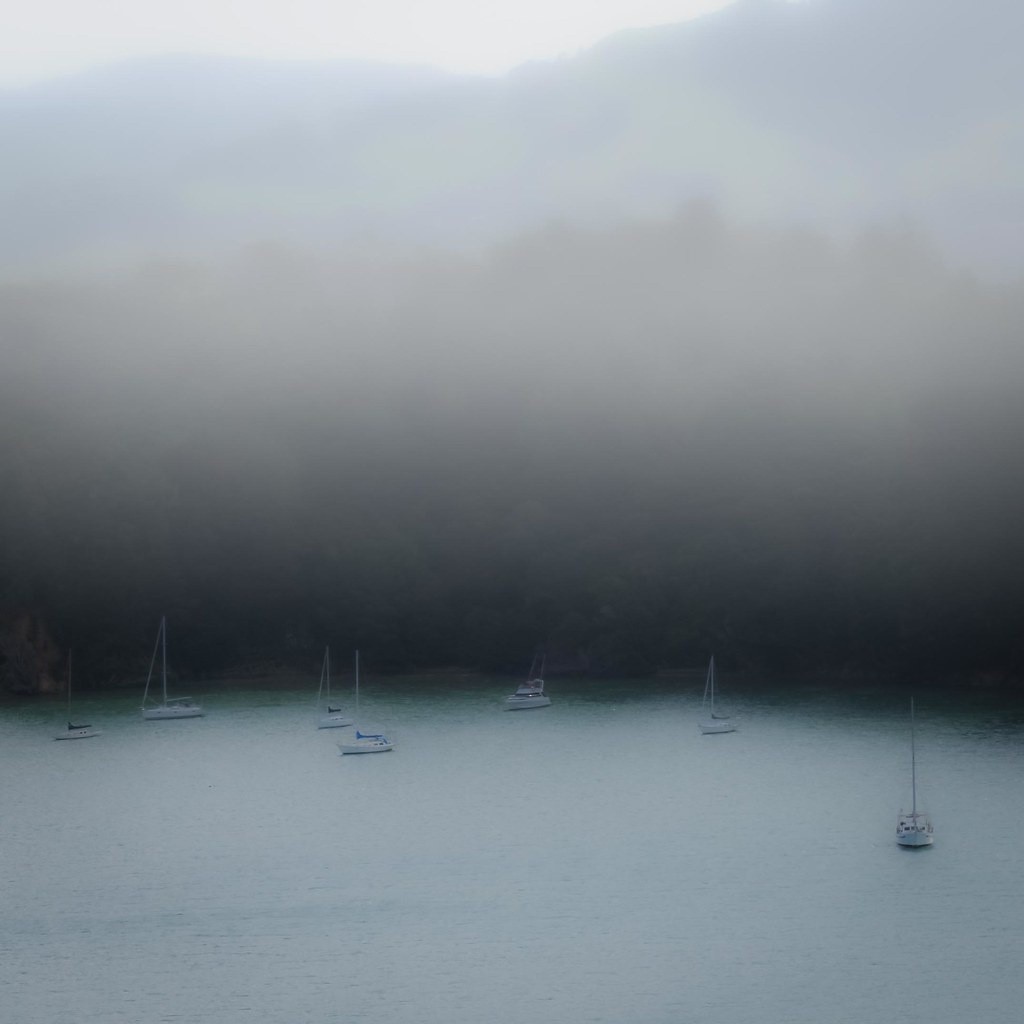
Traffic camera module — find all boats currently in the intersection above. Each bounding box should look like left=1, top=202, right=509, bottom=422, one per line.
left=54, top=730, right=100, bottom=740
left=68, top=721, right=93, bottom=729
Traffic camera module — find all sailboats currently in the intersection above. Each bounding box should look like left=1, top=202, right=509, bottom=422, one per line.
left=504, top=649, right=553, bottom=711
left=337, top=649, right=396, bottom=754
left=895, top=695, right=936, bottom=847
left=699, top=654, right=739, bottom=733
left=141, top=616, right=203, bottom=719
left=316, top=650, right=354, bottom=729
